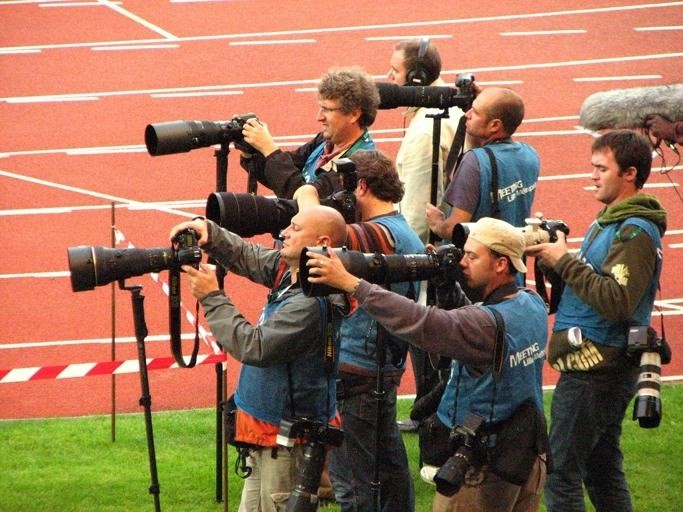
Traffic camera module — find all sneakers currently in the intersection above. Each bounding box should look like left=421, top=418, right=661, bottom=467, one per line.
left=396, top=417, right=422, bottom=432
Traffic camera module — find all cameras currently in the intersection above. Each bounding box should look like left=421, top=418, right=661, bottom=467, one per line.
left=410, top=367, right=450, bottom=421
left=433, top=424, right=500, bottom=497
left=276, top=418, right=345, bottom=512
left=626, top=324, right=671, bottom=428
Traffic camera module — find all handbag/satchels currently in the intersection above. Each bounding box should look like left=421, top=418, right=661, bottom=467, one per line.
left=485, top=395, right=551, bottom=491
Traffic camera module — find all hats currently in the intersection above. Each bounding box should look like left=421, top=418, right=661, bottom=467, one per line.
left=466, top=216, right=529, bottom=276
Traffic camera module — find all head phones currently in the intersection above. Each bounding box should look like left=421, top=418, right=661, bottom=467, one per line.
left=406, top=37, right=429, bottom=86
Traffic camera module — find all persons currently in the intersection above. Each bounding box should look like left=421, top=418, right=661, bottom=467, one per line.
left=525, top=131, right=667, bottom=511
left=170, top=41, right=549, bottom=512
